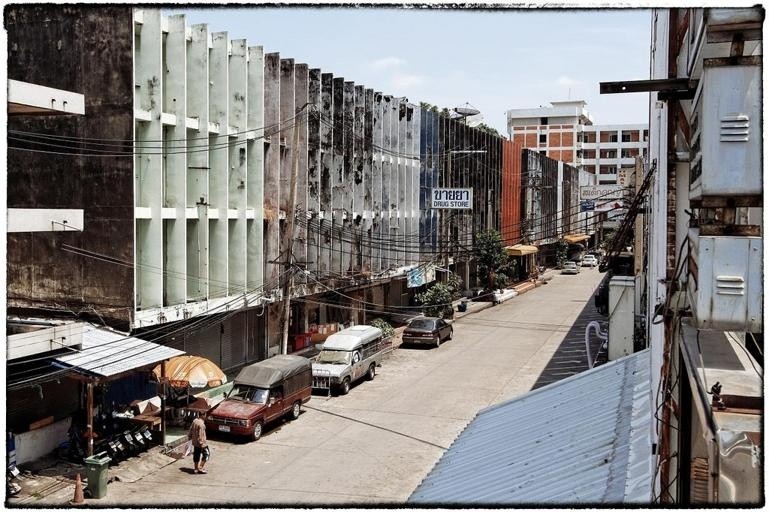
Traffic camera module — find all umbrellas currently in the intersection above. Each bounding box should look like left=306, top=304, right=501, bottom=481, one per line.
left=152, top=355, right=227, bottom=405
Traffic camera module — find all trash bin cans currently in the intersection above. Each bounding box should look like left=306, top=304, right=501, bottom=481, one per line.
left=83, top=451, right=112, bottom=499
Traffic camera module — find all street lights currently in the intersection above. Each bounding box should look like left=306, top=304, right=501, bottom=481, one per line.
left=280, top=264, right=310, bottom=355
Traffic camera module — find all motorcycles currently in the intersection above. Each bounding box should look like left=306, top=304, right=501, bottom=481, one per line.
left=66, top=412, right=154, bottom=466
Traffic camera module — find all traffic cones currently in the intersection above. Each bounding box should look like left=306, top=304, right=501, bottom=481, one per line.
left=69, top=473, right=88, bottom=505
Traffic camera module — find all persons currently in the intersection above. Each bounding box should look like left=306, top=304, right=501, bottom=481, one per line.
left=188, top=410, right=210, bottom=473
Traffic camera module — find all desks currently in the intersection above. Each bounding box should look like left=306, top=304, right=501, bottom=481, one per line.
left=132, top=414, right=162, bottom=431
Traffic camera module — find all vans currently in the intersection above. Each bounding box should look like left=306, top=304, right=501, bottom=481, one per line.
left=311, top=325, right=386, bottom=394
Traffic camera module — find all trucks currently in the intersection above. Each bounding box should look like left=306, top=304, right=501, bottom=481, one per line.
left=205, top=353, right=312, bottom=441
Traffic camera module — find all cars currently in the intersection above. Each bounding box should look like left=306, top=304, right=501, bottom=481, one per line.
left=402, top=315, right=452, bottom=348
left=581, top=252, right=602, bottom=268
left=561, top=262, right=580, bottom=273
